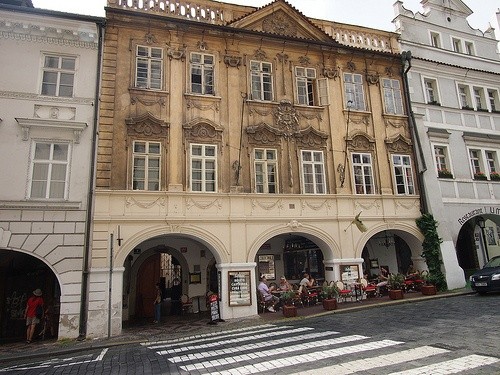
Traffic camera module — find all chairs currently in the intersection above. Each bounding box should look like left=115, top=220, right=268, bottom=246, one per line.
left=257, top=275, right=423, bottom=315
left=191, top=296, right=207, bottom=314
left=180, top=294, right=193, bottom=316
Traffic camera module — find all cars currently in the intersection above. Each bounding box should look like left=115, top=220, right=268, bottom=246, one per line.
left=469, top=255, right=500, bottom=295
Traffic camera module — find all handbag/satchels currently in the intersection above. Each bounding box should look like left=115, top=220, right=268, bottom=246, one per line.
left=36, top=298, right=43, bottom=319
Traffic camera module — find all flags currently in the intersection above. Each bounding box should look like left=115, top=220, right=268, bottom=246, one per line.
left=352, top=213, right=368, bottom=233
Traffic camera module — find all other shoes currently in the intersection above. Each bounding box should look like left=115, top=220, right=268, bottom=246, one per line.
left=267, top=308, right=276, bottom=313
left=26, top=338, right=32, bottom=344
left=153, top=320, right=160, bottom=323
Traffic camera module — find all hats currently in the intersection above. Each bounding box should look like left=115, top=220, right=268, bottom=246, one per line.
left=33, top=289, right=43, bottom=296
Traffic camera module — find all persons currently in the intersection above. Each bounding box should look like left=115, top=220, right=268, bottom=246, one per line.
left=358, top=273, right=369, bottom=288
left=258, top=278, right=279, bottom=312
left=25, top=289, right=44, bottom=345
left=376, top=267, right=389, bottom=289
left=152, top=283, right=163, bottom=325
left=278, top=276, right=291, bottom=292
left=300, top=274, right=314, bottom=291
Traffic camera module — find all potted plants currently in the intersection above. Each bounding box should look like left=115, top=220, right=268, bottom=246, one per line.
left=283, top=291, right=297, bottom=318
left=323, top=286, right=338, bottom=310
left=388, top=273, right=404, bottom=300
left=422, top=270, right=438, bottom=294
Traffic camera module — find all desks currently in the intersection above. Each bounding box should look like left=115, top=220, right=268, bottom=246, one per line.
left=272, top=291, right=285, bottom=294
left=308, top=286, right=322, bottom=290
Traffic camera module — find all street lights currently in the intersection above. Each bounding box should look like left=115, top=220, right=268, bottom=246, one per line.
left=478, top=217, right=490, bottom=261
